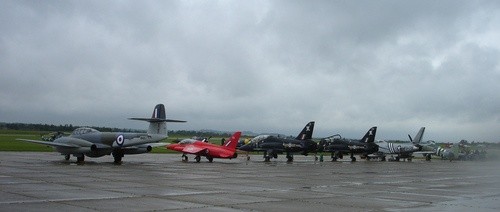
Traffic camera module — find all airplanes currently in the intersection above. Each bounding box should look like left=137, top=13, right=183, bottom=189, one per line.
left=165, top=131, right=244, bottom=162
left=222, top=121, right=437, bottom=163
left=15, top=104, right=187, bottom=165
